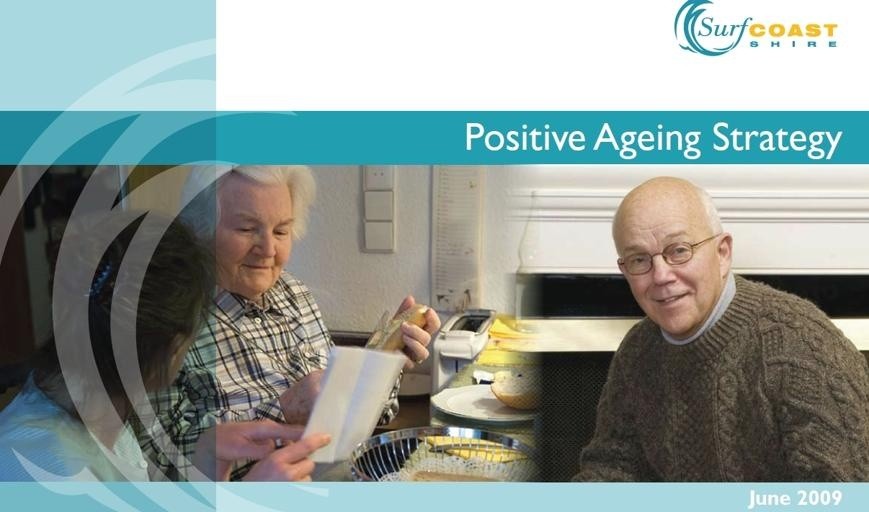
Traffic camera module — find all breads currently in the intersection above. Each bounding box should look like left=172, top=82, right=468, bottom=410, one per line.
left=364, top=304, right=431, bottom=351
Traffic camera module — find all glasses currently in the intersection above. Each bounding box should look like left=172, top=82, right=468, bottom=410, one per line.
left=616, top=232, right=723, bottom=277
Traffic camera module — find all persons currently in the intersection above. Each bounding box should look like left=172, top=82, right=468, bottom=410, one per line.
left=568, top=175, right=869, bottom=481
left=0, top=211, right=216, bottom=480
left=125, top=164, right=442, bottom=481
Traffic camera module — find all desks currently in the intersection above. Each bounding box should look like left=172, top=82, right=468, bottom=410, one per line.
left=396, top=326, right=541, bottom=481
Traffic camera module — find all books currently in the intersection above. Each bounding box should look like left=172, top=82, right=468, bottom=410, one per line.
left=297, top=345, right=410, bottom=466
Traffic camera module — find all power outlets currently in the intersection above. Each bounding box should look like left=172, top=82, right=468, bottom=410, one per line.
left=362, top=163, right=395, bottom=191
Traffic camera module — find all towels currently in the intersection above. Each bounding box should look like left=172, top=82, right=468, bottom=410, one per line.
left=418, top=432, right=528, bottom=464
left=475, top=312, right=532, bottom=369
